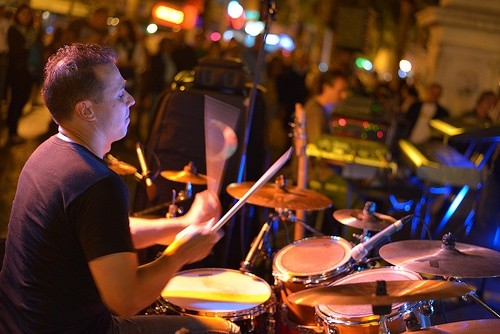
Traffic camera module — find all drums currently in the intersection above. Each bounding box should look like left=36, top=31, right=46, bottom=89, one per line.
left=158, top=267, right=276, bottom=334
left=273, top=233, right=356, bottom=328
left=316, top=266, right=431, bottom=334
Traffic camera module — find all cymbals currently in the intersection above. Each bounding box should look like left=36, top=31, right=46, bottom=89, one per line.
left=224, top=181, right=333, bottom=211
left=286, top=279, right=476, bottom=306
left=399, top=318, right=500, bottom=334
left=379, top=240, right=500, bottom=279
left=334, top=209, right=397, bottom=231
left=161, top=170, right=216, bottom=185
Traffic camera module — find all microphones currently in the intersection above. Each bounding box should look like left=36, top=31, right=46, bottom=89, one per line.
left=350, top=214, right=412, bottom=263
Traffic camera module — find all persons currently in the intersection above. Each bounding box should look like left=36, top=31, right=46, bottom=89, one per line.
left=0, top=44, right=224, bottom=334
left=0, top=0, right=500, bottom=228
